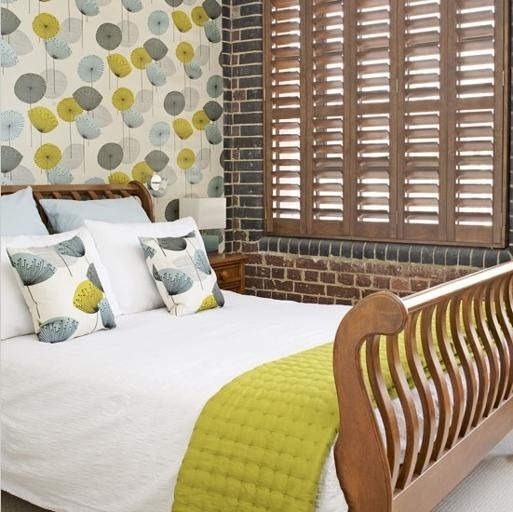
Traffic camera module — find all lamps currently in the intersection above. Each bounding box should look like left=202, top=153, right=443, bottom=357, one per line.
left=178, top=193, right=230, bottom=252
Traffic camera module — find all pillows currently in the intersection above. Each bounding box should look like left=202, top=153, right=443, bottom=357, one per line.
left=1, top=184, right=224, bottom=341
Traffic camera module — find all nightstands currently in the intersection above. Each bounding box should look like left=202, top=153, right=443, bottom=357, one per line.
left=207, top=252, right=250, bottom=293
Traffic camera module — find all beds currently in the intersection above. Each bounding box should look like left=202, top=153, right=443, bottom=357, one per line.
left=0, top=180, right=512, bottom=512
